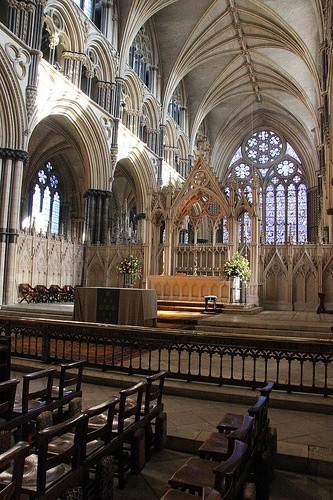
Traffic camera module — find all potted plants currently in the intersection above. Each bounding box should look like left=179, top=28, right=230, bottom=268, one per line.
left=117, top=251, right=143, bottom=287
left=221, top=249, right=250, bottom=303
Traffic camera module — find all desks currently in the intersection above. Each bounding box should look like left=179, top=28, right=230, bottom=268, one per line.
left=72, top=287, right=158, bottom=328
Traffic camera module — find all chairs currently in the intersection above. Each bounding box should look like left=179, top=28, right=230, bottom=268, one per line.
left=169, top=415, right=255, bottom=496
left=0, top=361, right=168, bottom=499
left=215, top=379, right=276, bottom=432
left=201, top=486, right=221, bottom=500
left=160, top=438, right=250, bottom=500
left=199, top=394, right=268, bottom=464
left=19, top=283, right=82, bottom=303
